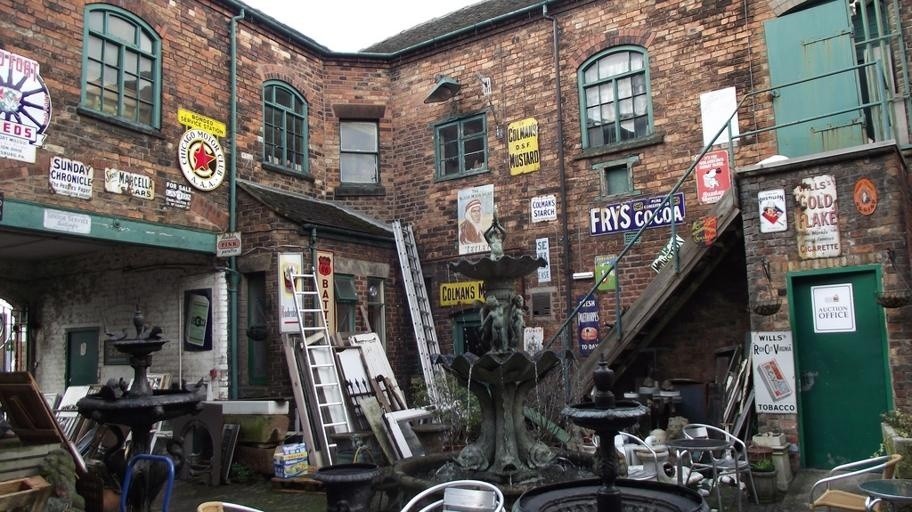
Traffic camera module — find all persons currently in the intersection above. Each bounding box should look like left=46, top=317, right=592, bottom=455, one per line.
left=459, top=200, right=485, bottom=245
left=480, top=295, right=527, bottom=351
left=485, top=222, right=506, bottom=261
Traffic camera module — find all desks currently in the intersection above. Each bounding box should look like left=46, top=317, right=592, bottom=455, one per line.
left=858, top=478, right=912, bottom=512
left=668, top=438, right=742, bottom=512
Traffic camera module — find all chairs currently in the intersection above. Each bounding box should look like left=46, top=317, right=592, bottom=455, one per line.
left=399, top=479, right=505, bottom=512
left=591, top=433, right=660, bottom=481
left=197, top=501, right=263, bottom=512
left=683, top=423, right=759, bottom=507
left=808, top=454, right=903, bottom=512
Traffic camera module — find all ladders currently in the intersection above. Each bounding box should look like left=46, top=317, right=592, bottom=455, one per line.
left=289, top=265, right=354, bottom=466
left=392, top=219, right=451, bottom=424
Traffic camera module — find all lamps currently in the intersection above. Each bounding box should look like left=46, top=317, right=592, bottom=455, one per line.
left=423, top=74, right=469, bottom=103
